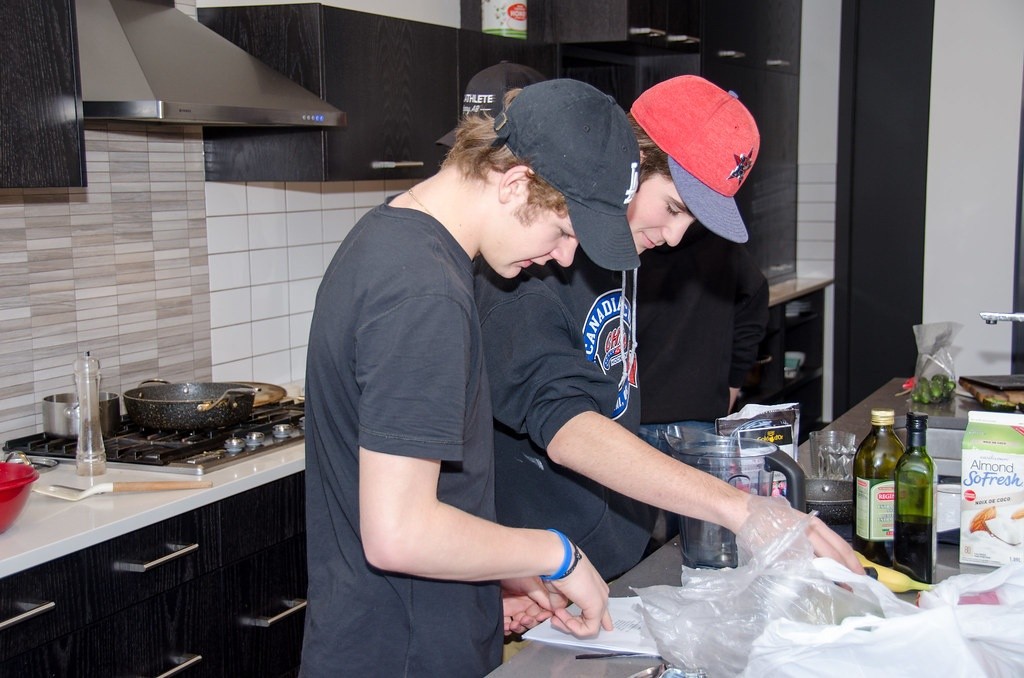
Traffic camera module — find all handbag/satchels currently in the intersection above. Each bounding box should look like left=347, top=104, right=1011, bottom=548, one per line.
left=627, top=493, right=1024, bottom=677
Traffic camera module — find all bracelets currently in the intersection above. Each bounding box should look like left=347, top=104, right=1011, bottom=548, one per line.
left=540, top=528, right=582, bottom=580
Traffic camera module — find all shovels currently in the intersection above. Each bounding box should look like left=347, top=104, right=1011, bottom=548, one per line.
left=32, top=481, right=213, bottom=502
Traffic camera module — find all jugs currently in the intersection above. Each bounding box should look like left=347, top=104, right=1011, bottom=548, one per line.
left=657, top=426, right=806, bottom=570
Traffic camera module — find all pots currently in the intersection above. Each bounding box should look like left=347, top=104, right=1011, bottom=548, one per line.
left=123, top=379, right=261, bottom=431
left=42, top=393, right=120, bottom=439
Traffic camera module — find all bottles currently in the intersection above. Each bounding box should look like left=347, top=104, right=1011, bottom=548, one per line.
left=853, top=409, right=906, bottom=567
left=72, top=351, right=108, bottom=477
left=893, top=413, right=938, bottom=585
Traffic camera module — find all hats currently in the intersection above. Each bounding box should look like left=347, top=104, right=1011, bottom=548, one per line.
left=493, top=77, right=641, bottom=271
left=435, top=60, right=547, bottom=148
left=631, top=75, right=760, bottom=240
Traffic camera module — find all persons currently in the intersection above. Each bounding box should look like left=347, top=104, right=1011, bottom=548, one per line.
left=299, top=80, right=641, bottom=678
left=473, top=76, right=866, bottom=592
left=641, top=219, right=773, bottom=547
left=439, top=62, right=531, bottom=143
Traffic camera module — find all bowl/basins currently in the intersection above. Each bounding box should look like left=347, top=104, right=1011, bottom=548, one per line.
left=0, top=453, right=39, bottom=534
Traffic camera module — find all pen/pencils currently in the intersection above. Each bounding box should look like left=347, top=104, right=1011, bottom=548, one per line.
left=576, top=653, right=635, bottom=659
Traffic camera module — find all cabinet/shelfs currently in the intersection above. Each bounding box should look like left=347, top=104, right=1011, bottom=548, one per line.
left=0, top=0, right=88, bottom=188
left=0, top=469, right=307, bottom=678
left=197, top=0, right=543, bottom=182
left=537, top=0, right=701, bottom=53
left=699, top=0, right=802, bottom=287
left=767, top=288, right=827, bottom=441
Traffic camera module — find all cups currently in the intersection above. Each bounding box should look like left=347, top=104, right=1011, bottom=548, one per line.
left=811, top=431, right=856, bottom=481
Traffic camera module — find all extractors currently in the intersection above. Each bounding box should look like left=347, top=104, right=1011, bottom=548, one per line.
left=72, top=0, right=346, bottom=126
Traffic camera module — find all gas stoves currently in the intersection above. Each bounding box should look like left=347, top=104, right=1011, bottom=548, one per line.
left=4, top=395, right=306, bottom=476
